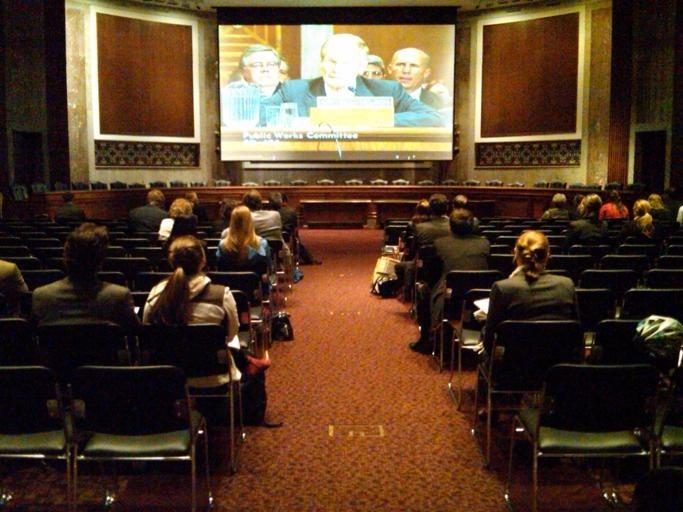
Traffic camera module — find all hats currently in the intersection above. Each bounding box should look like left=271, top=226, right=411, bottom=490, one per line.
left=368, top=55, right=385, bottom=69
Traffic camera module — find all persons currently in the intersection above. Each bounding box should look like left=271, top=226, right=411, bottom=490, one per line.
left=395, top=194, right=478, bottom=297
left=54, top=191, right=87, bottom=221
left=32, top=223, right=143, bottom=367
left=132, top=189, right=323, bottom=306
left=540, top=190, right=683, bottom=251
left=222, top=33, right=452, bottom=128
left=142, top=235, right=243, bottom=427
left=408, top=208, right=490, bottom=354
left=0, top=258, right=28, bottom=317
left=470, top=229, right=580, bottom=404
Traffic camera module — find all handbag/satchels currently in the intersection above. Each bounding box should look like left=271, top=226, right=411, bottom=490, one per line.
left=235, top=349, right=272, bottom=427
left=272, top=312, right=294, bottom=341
left=373, top=257, right=399, bottom=298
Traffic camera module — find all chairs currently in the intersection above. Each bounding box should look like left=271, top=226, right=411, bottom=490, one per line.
left=3, top=175, right=683, bottom=228
left=400, top=213, right=682, bottom=510
left=2, top=193, right=301, bottom=511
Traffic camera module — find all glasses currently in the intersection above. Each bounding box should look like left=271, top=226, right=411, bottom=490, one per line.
left=363, top=72, right=382, bottom=76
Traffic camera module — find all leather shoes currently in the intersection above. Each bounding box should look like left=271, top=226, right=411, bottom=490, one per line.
left=410, top=341, right=430, bottom=351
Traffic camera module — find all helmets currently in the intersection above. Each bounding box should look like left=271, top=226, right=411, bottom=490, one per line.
left=631, top=315, right=681, bottom=357
